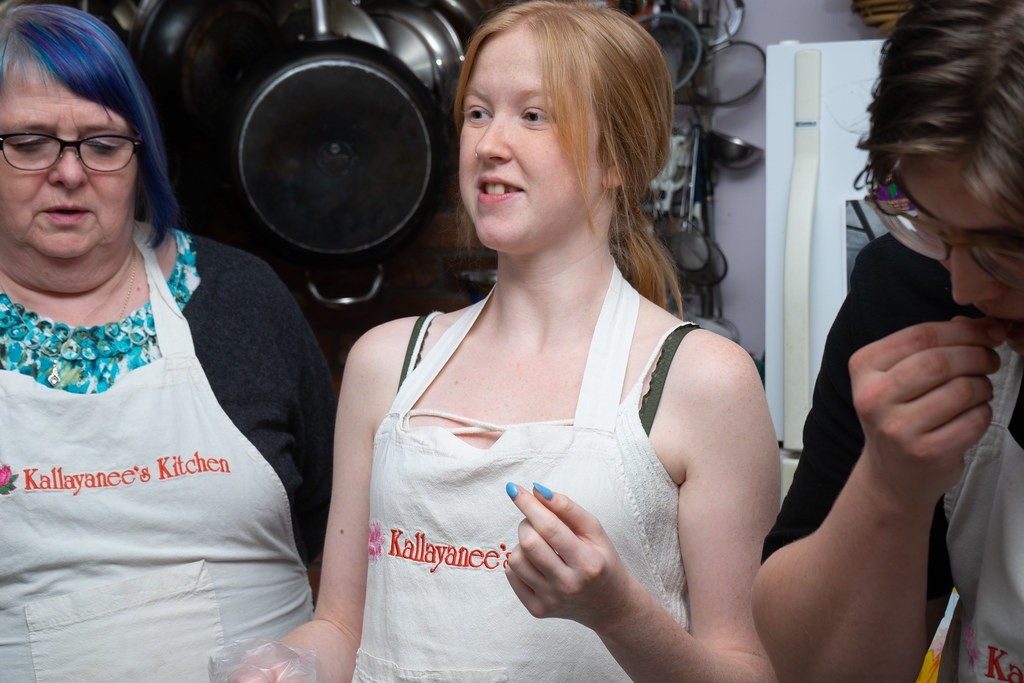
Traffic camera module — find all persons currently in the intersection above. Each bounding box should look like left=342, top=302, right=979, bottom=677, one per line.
left=0, top=3, right=347, bottom=683
left=742, top=0, right=1024, bottom=683
left=233, top=0, right=780, bottom=682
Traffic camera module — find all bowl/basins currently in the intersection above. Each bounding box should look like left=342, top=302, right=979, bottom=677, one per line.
left=850, top=0, right=915, bottom=38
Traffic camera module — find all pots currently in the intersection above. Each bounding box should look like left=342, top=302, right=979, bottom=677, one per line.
left=68, top=0, right=504, bottom=311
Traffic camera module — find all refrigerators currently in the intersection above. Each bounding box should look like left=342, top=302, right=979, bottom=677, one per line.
left=762, top=39, right=962, bottom=682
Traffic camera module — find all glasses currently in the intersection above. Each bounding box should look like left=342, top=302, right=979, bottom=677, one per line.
left=0, top=130, right=141, bottom=172
left=862, top=190, right=1023, bottom=291
